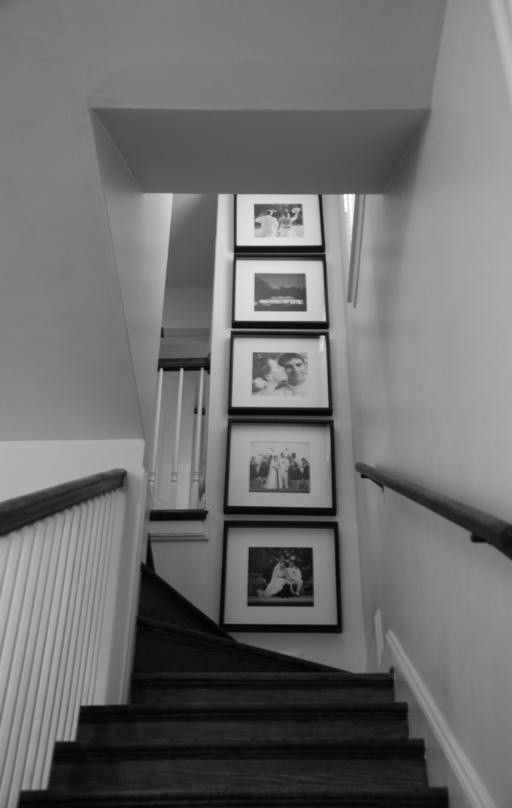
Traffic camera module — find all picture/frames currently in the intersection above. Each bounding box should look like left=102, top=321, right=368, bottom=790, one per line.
left=223, top=416, right=336, bottom=516
left=234, top=194, right=324, bottom=251
left=229, top=328, right=335, bottom=416
left=232, top=254, right=331, bottom=328
left=219, top=518, right=345, bottom=635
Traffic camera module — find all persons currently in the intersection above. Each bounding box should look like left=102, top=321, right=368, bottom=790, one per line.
left=255, top=208, right=297, bottom=237
left=257, top=549, right=304, bottom=598
left=253, top=353, right=308, bottom=396
left=250, top=452, right=310, bottom=491
